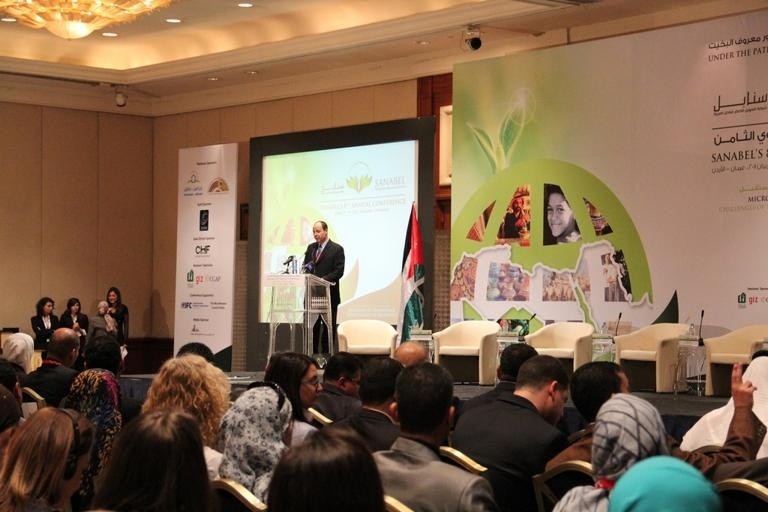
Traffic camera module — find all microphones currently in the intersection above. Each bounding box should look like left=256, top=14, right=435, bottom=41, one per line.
left=283, top=255, right=296, bottom=265
left=518, top=313, right=537, bottom=341
left=428, top=313, right=437, bottom=330
left=612, top=312, right=623, bottom=344
left=698, top=310, right=704, bottom=346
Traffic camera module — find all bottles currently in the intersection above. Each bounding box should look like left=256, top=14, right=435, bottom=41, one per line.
left=411, top=319, right=420, bottom=334
left=502, top=319, right=509, bottom=335
left=291, top=255, right=297, bottom=275
left=688, top=324, right=696, bottom=338
left=599, top=322, right=608, bottom=335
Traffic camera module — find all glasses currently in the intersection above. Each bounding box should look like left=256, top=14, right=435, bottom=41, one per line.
left=300, top=378, right=319, bottom=386
left=247, top=381, right=286, bottom=412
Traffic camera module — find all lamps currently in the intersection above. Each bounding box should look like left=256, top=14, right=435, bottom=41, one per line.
left=461, top=25, right=482, bottom=51
left=0, top=0, right=172, bottom=39
left=115, top=84, right=128, bottom=107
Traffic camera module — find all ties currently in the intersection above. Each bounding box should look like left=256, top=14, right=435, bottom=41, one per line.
left=316, top=244, right=321, bottom=260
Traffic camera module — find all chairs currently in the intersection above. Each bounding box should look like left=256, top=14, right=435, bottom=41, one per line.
left=532, top=459, right=596, bottom=511
left=524, top=321, right=595, bottom=373
left=432, top=320, right=503, bottom=385
left=305, top=407, right=334, bottom=432
left=375, top=443, right=488, bottom=484
left=384, top=494, right=416, bottom=512
left=338, top=319, right=398, bottom=359
left=715, top=477, right=766, bottom=511
left=703, top=325, right=768, bottom=396
left=614, top=322, right=689, bottom=393
left=210, top=477, right=268, bottom=510
left=20, top=385, right=46, bottom=412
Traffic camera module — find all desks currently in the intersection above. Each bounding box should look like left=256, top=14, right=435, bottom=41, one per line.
left=680, top=336, right=706, bottom=396
left=591, top=331, right=614, bottom=355
left=406, top=337, right=433, bottom=364
left=497, top=335, right=524, bottom=361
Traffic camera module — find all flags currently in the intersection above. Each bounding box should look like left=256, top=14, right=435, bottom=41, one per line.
left=395, top=202, right=426, bottom=351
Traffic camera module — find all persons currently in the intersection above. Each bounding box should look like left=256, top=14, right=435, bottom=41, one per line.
left=503, top=192, right=529, bottom=237
left=1, top=327, right=768, bottom=511
left=31, top=287, right=130, bottom=375
left=547, top=189, right=582, bottom=244
left=301, top=220, right=344, bottom=356
